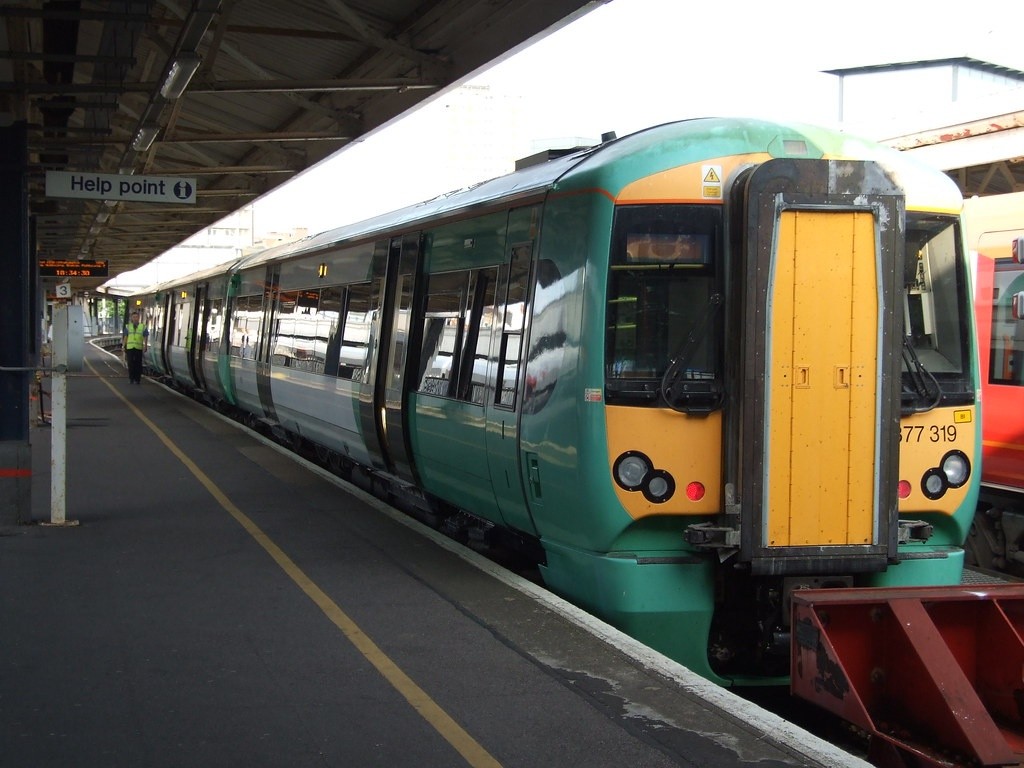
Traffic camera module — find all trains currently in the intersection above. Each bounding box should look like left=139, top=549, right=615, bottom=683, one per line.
left=875, top=109, right=1024, bottom=578
left=122, top=114, right=985, bottom=690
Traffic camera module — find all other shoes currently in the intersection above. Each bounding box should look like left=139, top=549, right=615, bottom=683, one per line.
left=129, top=379, right=134, bottom=384
left=135, top=379, right=140, bottom=384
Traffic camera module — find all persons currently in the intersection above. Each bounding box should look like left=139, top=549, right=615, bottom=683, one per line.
left=122, top=312, right=148, bottom=384
